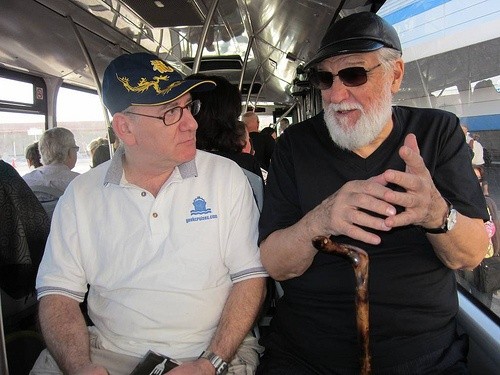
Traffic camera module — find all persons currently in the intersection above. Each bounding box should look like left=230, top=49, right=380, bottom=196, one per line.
left=22, top=127, right=81, bottom=219
left=90, top=144, right=110, bottom=168
left=257, top=11, right=489, bottom=375
left=455, top=164, right=500, bottom=308
left=237, top=120, right=268, bottom=186
left=24, top=141, right=43, bottom=173
left=28, top=52, right=269, bottom=375
left=185, top=75, right=266, bottom=191
left=278, top=118, right=289, bottom=134
left=88, top=137, right=108, bottom=156
left=260, top=128, right=277, bottom=142
left=242, top=111, right=275, bottom=170
left=460, top=122, right=486, bottom=176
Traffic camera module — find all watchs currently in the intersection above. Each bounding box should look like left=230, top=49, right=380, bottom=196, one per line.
left=198, top=351, right=228, bottom=375
left=419, top=196, right=456, bottom=234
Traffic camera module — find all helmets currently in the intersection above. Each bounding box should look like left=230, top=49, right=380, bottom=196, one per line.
left=185, top=72, right=241, bottom=129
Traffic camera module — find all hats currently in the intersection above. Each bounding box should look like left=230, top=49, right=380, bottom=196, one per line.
left=102, top=53, right=216, bottom=117
left=302, top=11, right=402, bottom=71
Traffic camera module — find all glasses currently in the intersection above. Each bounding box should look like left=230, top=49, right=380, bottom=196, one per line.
left=73, top=145, right=79, bottom=152
left=311, top=63, right=382, bottom=90
left=126, top=99, right=201, bottom=126
